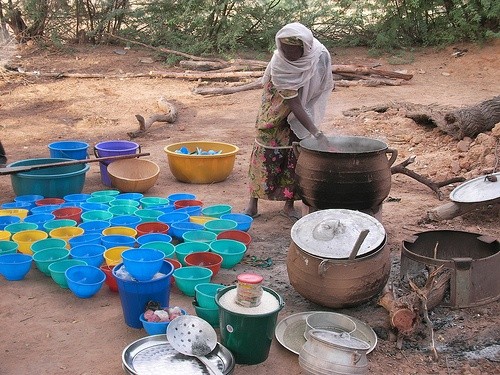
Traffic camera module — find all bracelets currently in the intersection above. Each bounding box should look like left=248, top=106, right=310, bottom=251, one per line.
left=314, top=131, right=323, bottom=138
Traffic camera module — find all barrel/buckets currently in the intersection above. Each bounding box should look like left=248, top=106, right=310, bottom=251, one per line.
left=48, top=141, right=90, bottom=164
left=111, top=262, right=175, bottom=329
left=215, top=282, right=284, bottom=364
left=93, top=141, right=142, bottom=187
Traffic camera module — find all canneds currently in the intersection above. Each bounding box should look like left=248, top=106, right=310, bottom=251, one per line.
left=235, top=273, right=263, bottom=307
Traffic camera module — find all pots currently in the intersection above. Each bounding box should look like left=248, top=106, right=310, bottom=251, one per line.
left=298, top=330, right=369, bottom=375
left=287, top=212, right=395, bottom=311
left=290, top=134, right=397, bottom=214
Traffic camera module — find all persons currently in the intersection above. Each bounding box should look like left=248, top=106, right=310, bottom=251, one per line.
left=244, top=22, right=334, bottom=221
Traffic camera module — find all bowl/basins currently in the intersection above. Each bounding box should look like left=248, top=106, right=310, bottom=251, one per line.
left=303, top=311, right=357, bottom=342
left=7, top=159, right=90, bottom=198
left=0, top=190, right=253, bottom=323
left=165, top=141, right=240, bottom=184
left=139, top=309, right=188, bottom=336
left=106, top=159, right=161, bottom=195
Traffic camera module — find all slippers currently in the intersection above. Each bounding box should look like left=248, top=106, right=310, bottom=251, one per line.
left=245, top=212, right=260, bottom=218
left=280, top=209, right=302, bottom=223
